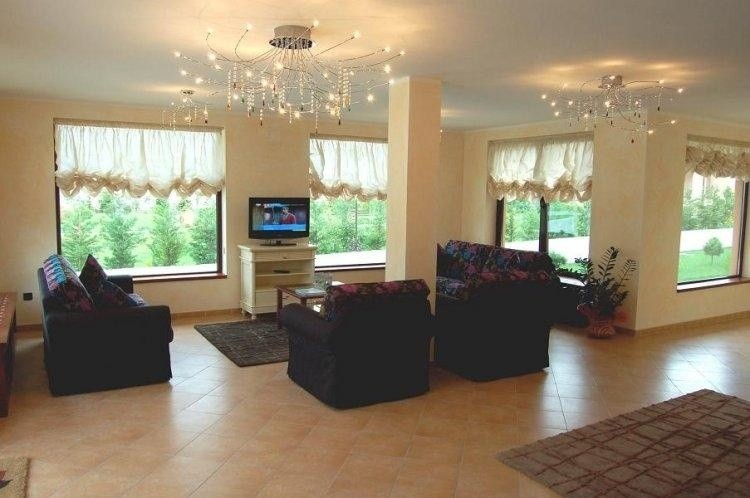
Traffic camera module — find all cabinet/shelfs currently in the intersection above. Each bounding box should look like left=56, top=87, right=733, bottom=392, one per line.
left=238, top=243, right=318, bottom=320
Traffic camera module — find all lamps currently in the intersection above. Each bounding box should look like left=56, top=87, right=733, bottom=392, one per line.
left=540, top=72, right=689, bottom=145
left=171, top=20, right=405, bottom=134
left=160, top=89, right=209, bottom=134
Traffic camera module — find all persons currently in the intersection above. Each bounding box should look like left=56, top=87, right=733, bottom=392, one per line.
left=278, top=206, right=297, bottom=225
left=263, top=210, right=274, bottom=225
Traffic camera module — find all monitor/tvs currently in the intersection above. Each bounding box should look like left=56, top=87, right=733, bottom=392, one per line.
left=248, top=197, right=310, bottom=246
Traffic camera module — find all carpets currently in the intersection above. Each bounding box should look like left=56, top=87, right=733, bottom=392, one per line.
left=0, top=456, right=29, bottom=498
left=194, top=316, right=292, bottom=367
left=493, top=388, right=750, bottom=497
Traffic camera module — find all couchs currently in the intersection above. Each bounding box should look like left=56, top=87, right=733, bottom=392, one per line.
left=36, top=255, right=174, bottom=397
left=434, top=239, right=559, bottom=383
left=279, top=277, right=432, bottom=409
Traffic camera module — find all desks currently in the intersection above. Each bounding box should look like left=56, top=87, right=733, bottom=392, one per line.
left=0, top=292, right=19, bottom=415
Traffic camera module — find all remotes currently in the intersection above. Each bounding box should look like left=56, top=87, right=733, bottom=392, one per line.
left=273, top=269, right=290, bottom=273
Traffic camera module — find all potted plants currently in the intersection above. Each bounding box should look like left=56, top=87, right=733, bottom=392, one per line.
left=554, top=247, right=637, bottom=338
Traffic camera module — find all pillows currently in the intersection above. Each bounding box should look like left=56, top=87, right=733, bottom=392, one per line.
left=43, top=255, right=94, bottom=310
left=92, top=280, right=139, bottom=308
left=78, top=254, right=107, bottom=294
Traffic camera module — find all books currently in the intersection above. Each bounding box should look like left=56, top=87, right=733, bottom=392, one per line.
left=295, top=286, right=326, bottom=298
